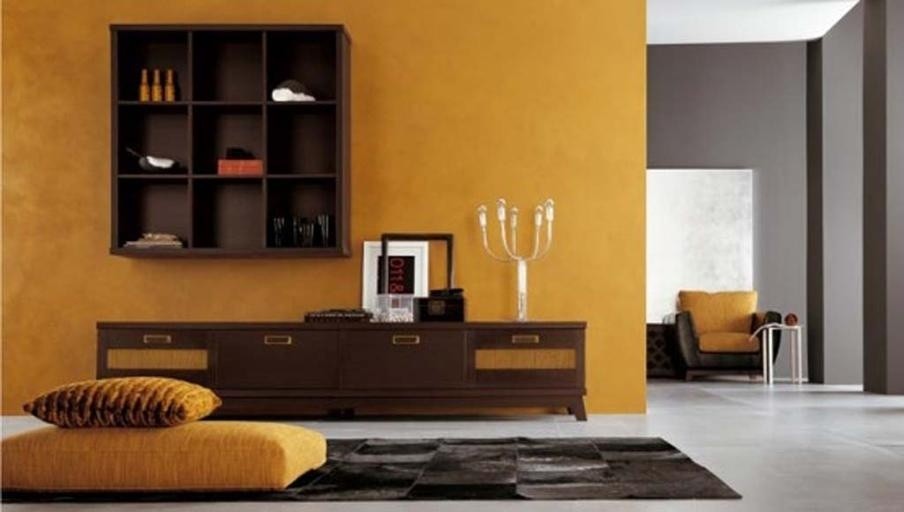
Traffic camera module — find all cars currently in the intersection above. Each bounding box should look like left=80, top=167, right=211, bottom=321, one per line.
left=272, top=214, right=334, bottom=249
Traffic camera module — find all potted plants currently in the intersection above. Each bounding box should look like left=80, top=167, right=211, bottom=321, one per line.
left=359, top=240, right=430, bottom=320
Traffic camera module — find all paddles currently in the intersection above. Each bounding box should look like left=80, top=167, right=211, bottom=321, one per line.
left=1, top=434, right=742, bottom=503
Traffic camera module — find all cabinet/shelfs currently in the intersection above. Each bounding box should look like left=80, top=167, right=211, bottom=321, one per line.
left=139, top=68, right=179, bottom=101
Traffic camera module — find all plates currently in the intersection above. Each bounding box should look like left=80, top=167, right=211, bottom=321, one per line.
left=104, top=22, right=352, bottom=261
left=93, top=320, right=587, bottom=425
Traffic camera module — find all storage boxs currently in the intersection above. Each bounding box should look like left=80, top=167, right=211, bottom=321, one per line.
left=1, top=415, right=327, bottom=491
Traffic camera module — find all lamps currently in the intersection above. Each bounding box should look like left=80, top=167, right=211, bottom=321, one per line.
left=672, top=290, right=784, bottom=384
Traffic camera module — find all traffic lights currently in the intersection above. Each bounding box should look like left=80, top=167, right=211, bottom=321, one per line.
left=16, top=374, right=223, bottom=428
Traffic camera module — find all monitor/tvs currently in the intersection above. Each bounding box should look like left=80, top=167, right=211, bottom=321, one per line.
left=123, top=240, right=183, bottom=248
